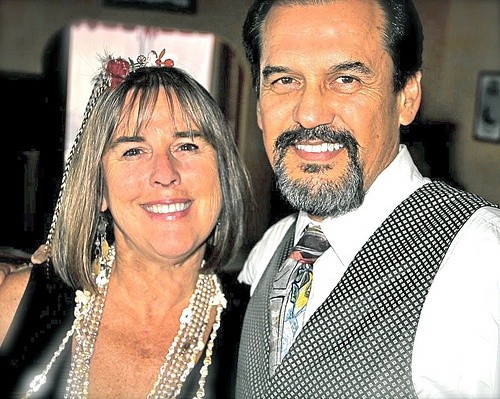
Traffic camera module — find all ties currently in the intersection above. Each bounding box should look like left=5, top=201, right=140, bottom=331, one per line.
left=267, top=224, right=331, bottom=379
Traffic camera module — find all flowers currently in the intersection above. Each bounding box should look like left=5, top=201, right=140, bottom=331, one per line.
left=102, top=48, right=174, bottom=87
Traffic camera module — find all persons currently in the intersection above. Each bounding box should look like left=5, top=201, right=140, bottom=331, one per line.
left=0, top=0, right=500, bottom=399
left=0, top=49, right=259, bottom=399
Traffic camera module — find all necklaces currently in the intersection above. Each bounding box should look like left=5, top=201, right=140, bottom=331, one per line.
left=24, top=242, right=228, bottom=399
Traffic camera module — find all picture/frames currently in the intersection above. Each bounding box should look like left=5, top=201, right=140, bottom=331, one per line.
left=472, top=69, right=500, bottom=143
